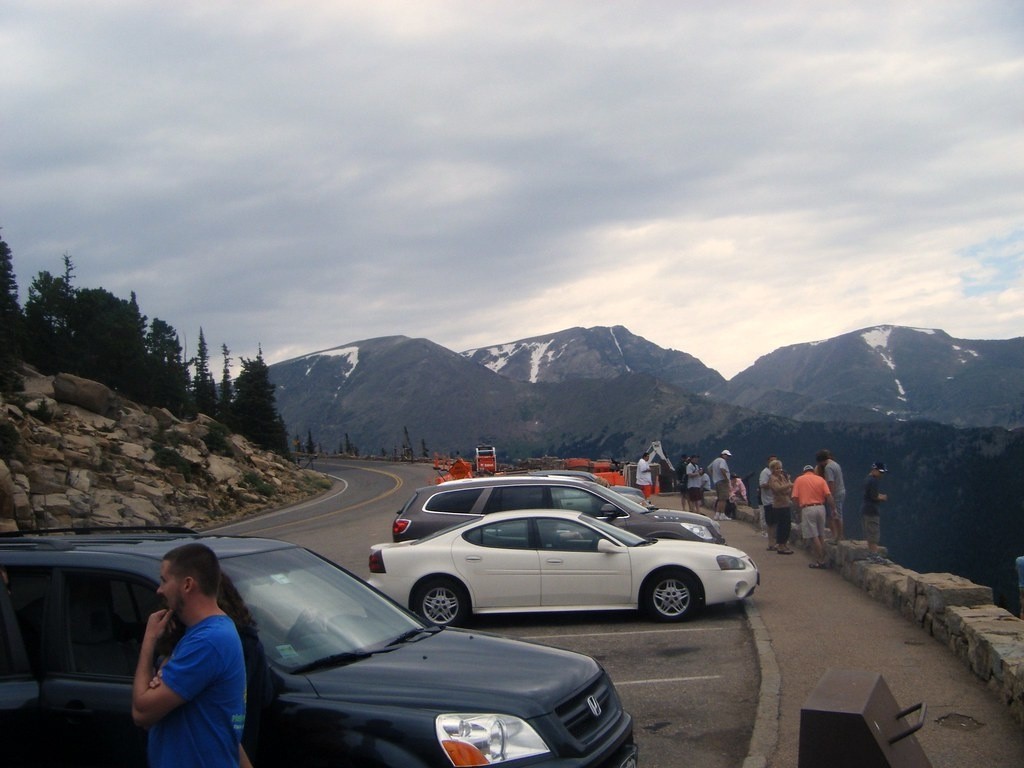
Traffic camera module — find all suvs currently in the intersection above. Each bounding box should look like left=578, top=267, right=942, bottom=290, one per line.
left=0, top=524, right=637, bottom=768
left=391, top=473, right=728, bottom=545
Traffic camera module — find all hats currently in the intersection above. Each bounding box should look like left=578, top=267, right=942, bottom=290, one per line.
left=691, top=454, right=700, bottom=458
left=803, top=465, right=815, bottom=471
left=872, top=460, right=888, bottom=473
left=721, top=450, right=732, bottom=455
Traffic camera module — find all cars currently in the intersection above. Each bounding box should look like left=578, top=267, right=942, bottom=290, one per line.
left=368, top=508, right=761, bottom=632
left=485, top=468, right=657, bottom=510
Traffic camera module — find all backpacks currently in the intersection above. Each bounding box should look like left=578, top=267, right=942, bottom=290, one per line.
left=675, top=461, right=686, bottom=478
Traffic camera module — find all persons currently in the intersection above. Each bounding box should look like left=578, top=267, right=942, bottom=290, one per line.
left=635, top=453, right=654, bottom=504
left=591, top=477, right=610, bottom=510
left=759, top=449, right=846, bottom=568
left=0, top=566, right=42, bottom=677
left=541, top=522, right=577, bottom=542
left=1016, top=556, right=1024, bottom=620
left=859, top=462, right=890, bottom=553
left=676, top=454, right=711, bottom=516
left=131, top=543, right=275, bottom=768
left=707, top=449, right=748, bottom=521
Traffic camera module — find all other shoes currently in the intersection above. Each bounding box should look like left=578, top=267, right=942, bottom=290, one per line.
left=808, top=560, right=827, bottom=568
left=767, top=545, right=778, bottom=550
left=828, top=538, right=844, bottom=545
left=867, top=552, right=887, bottom=564
left=719, top=515, right=732, bottom=521
left=714, top=515, right=720, bottom=520
left=777, top=547, right=793, bottom=554
